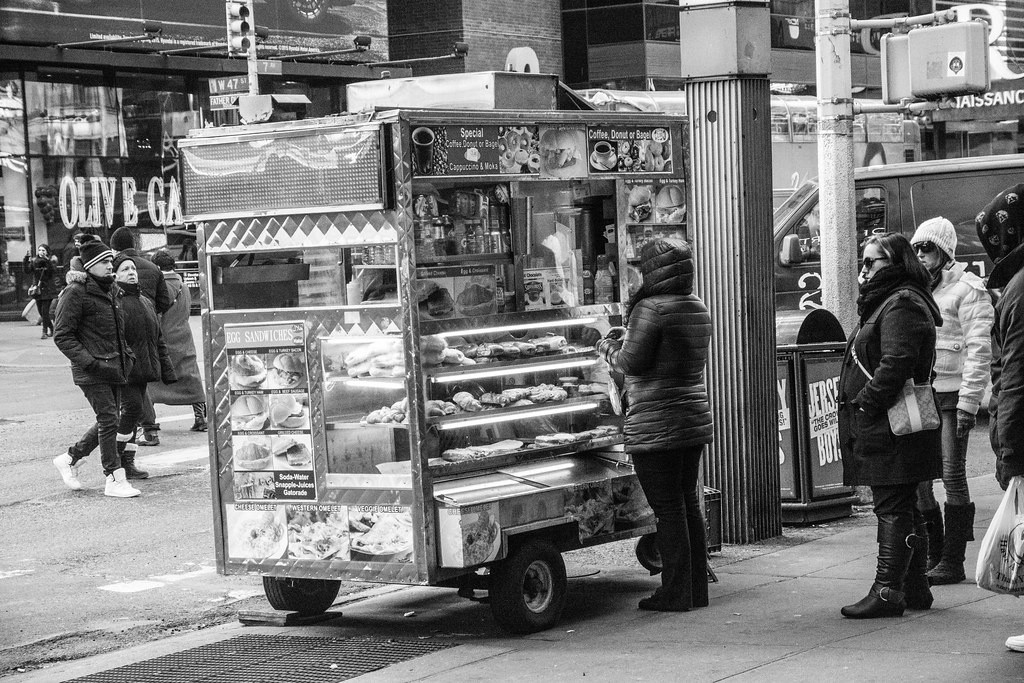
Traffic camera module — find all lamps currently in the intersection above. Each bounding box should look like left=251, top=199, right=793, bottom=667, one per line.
left=158, top=25, right=269, bottom=55
left=57, top=24, right=162, bottom=48
left=268, top=35, right=372, bottom=60
left=365, top=42, right=469, bottom=67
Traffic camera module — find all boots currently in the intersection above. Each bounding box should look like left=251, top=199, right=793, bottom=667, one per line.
left=903, top=522, right=933, bottom=611
left=122, top=443, right=148, bottom=479
left=116, top=432, right=134, bottom=459
left=922, top=502, right=942, bottom=573
left=842, top=515, right=915, bottom=618
left=925, top=502, right=975, bottom=582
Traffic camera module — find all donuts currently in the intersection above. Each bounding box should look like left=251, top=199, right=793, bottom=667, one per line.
left=618, top=141, right=672, bottom=172
left=497, top=126, right=532, bottom=156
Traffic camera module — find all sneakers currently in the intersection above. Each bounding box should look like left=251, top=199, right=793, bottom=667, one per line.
left=104, top=468, right=140, bottom=498
left=53, top=452, right=86, bottom=490
left=190, top=417, right=208, bottom=432
left=136, top=426, right=160, bottom=446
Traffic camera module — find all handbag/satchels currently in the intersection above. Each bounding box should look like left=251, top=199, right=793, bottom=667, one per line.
left=887, top=377, right=939, bottom=435
left=28, top=287, right=41, bottom=296
left=975, top=476, right=1024, bottom=594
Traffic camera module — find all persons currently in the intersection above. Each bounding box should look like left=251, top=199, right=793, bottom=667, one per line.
left=54, top=243, right=141, bottom=497
left=910, top=216, right=994, bottom=586
left=178, top=239, right=198, bottom=269
left=112, top=256, right=178, bottom=479
left=110, top=226, right=207, bottom=446
left=596, top=238, right=714, bottom=610
left=840, top=231, right=944, bottom=618
left=29, top=231, right=101, bottom=339
left=974, top=183, right=1024, bottom=654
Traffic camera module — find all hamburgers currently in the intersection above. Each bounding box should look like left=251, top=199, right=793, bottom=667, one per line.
left=628, top=186, right=653, bottom=221
left=230, top=352, right=306, bottom=430
left=658, top=185, right=686, bottom=224
left=541, top=130, right=578, bottom=177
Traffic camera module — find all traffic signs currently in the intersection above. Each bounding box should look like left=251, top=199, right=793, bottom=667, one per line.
left=208, top=75, right=249, bottom=93
left=209, top=94, right=248, bottom=111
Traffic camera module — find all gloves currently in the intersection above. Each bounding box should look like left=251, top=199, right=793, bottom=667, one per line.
left=956, top=409, right=976, bottom=437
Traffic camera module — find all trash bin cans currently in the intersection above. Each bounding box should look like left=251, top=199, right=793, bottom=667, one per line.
left=776, top=309, right=857, bottom=525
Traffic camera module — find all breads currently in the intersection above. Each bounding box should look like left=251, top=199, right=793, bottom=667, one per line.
left=417, top=280, right=494, bottom=316
left=343, top=336, right=618, bottom=461
left=236, top=438, right=309, bottom=469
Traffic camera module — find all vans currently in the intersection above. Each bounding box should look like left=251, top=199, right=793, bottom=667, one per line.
left=773, top=153, right=1024, bottom=313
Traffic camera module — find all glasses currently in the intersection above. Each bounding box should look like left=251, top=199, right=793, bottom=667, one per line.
left=913, top=241, right=936, bottom=254
left=862, top=256, right=889, bottom=268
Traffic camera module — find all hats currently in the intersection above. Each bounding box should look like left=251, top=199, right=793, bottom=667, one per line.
left=111, top=254, right=135, bottom=279
left=77, top=234, right=113, bottom=271
left=110, top=227, right=136, bottom=251
left=910, top=217, right=958, bottom=260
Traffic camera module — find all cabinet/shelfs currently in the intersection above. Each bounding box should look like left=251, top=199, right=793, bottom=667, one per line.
left=325, top=302, right=628, bottom=484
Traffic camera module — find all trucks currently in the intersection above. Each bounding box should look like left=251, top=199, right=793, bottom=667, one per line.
left=178, top=67, right=696, bottom=634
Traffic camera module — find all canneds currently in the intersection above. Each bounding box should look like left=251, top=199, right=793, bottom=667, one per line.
left=490, top=231, right=501, bottom=253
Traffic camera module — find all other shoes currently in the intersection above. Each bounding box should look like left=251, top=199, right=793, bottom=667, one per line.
left=1005, top=633, right=1024, bottom=652
left=48, top=325, right=54, bottom=337
left=638, top=591, right=692, bottom=612
left=692, top=598, right=709, bottom=608
left=41, top=327, right=47, bottom=338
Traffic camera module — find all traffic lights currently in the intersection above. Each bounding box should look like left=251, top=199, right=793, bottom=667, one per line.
left=226, top=1, right=251, bottom=57
